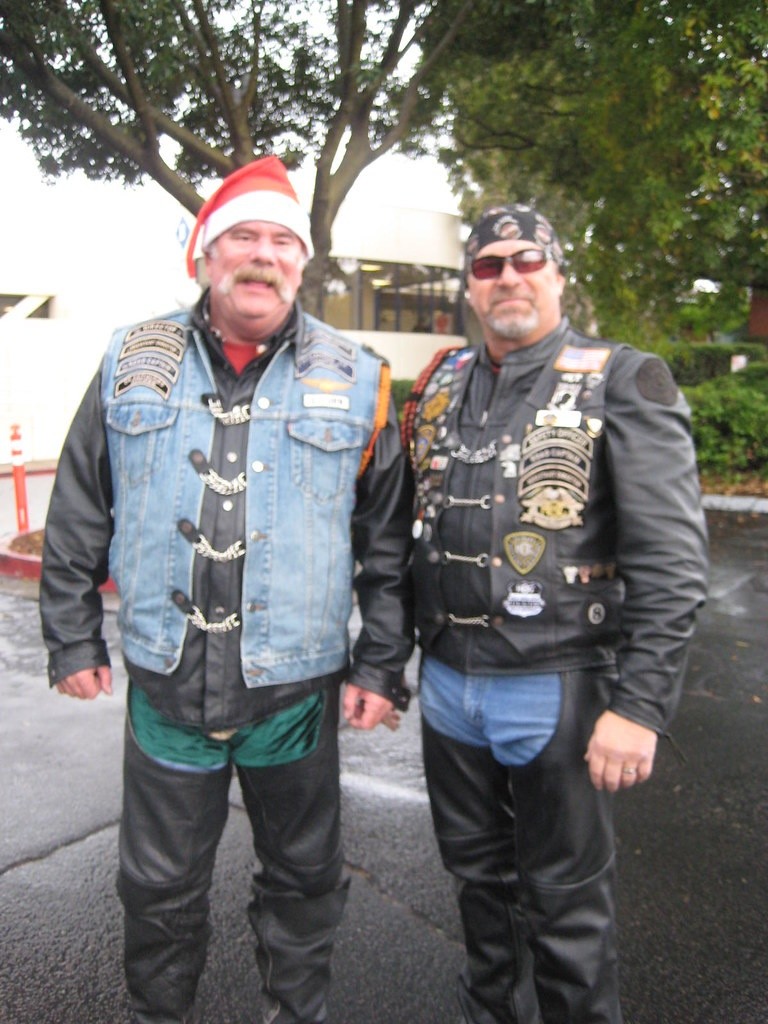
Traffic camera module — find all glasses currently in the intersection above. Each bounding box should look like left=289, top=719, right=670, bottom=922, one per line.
left=469, top=249, right=556, bottom=279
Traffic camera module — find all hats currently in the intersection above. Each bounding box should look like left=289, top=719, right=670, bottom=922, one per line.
left=187, top=155, right=315, bottom=279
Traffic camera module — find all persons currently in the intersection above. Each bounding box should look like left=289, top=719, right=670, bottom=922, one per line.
left=382, top=214, right=709, bottom=1024
left=39, top=157, right=412, bottom=1024
